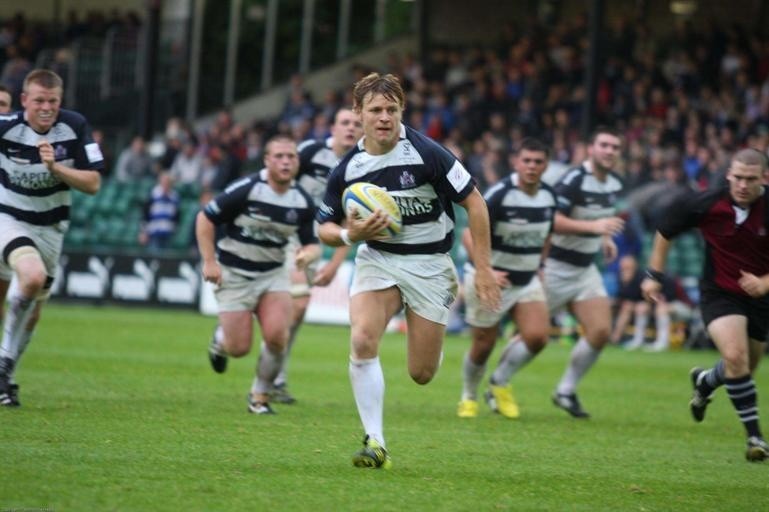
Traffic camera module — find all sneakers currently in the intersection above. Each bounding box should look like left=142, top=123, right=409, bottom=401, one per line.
left=688, top=365, right=712, bottom=421
left=209, top=324, right=230, bottom=373
left=353, top=434, right=390, bottom=467
left=248, top=393, right=273, bottom=413
left=1, top=369, right=22, bottom=407
left=552, top=385, right=591, bottom=418
left=271, top=381, right=295, bottom=405
left=485, top=380, right=522, bottom=418
left=746, top=435, right=768, bottom=460
left=456, top=397, right=478, bottom=419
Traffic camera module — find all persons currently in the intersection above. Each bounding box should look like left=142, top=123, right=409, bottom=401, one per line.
left=318, top=75, right=500, bottom=469
left=196, top=105, right=364, bottom=414
left=136, top=1, right=766, bottom=352
left=0, top=69, right=103, bottom=408
left=2, top=6, right=185, bottom=190
left=456, top=122, right=627, bottom=422
left=647, top=150, right=769, bottom=464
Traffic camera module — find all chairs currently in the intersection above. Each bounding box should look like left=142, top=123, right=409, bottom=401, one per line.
left=65, top=176, right=199, bottom=249
left=642, top=226, right=704, bottom=278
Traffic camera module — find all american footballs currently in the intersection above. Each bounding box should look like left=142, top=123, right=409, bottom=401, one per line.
left=342, top=182, right=403, bottom=238
